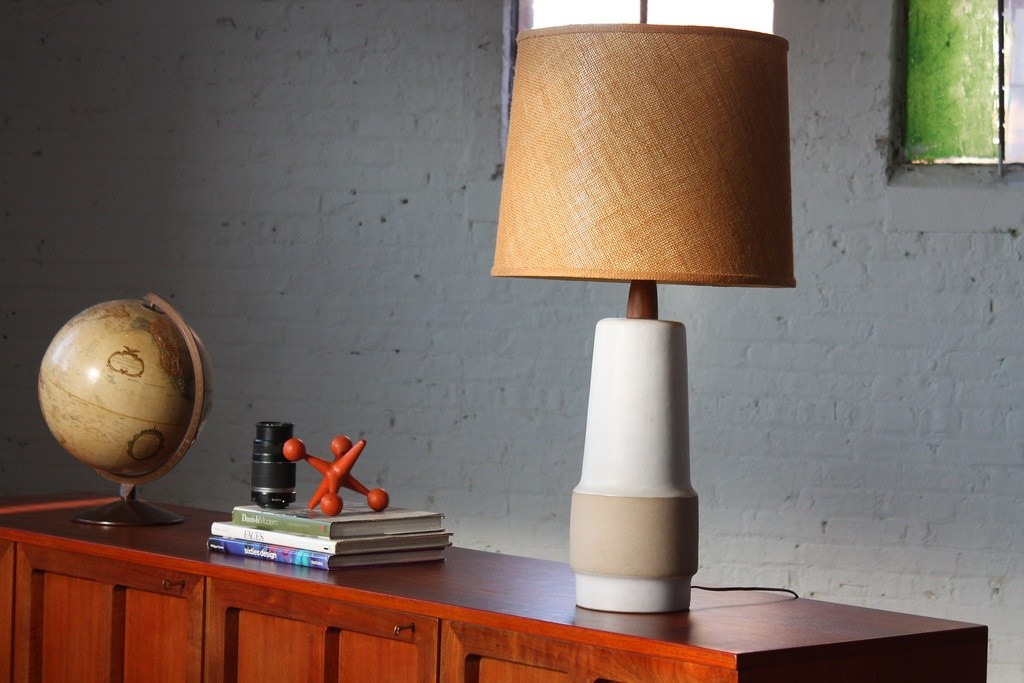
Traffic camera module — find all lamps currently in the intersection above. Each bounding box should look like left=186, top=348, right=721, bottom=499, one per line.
left=489, top=22, right=797, bottom=616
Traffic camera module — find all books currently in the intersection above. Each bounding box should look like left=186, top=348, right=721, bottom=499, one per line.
left=207, top=501, right=453, bottom=571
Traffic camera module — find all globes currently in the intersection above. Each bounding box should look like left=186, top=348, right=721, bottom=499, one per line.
left=37, top=292, right=215, bottom=526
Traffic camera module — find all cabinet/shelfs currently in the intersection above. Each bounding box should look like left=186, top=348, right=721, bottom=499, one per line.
left=0, top=491, right=989, bottom=683
